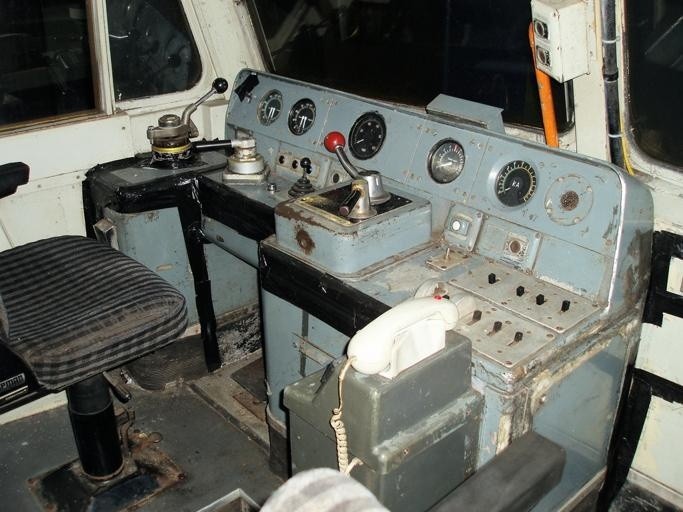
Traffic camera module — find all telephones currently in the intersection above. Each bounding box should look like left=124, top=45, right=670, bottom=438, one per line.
left=347, top=296, right=458, bottom=379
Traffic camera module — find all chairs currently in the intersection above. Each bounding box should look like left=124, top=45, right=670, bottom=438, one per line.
left=0, top=162, right=189, bottom=512
